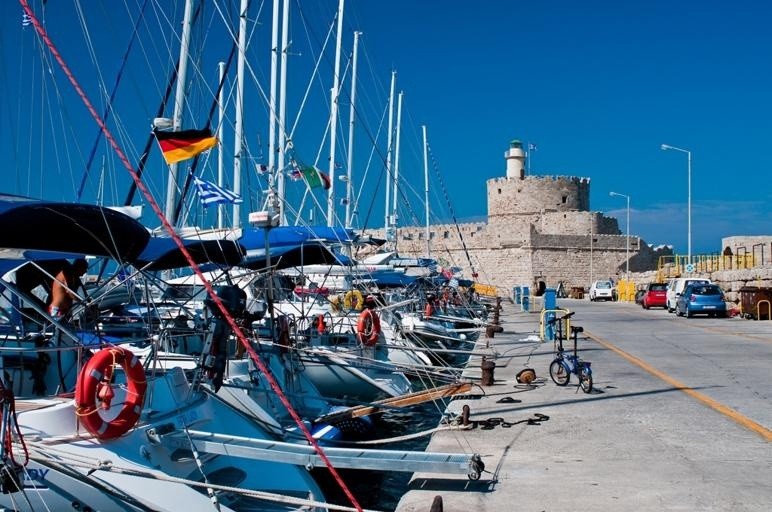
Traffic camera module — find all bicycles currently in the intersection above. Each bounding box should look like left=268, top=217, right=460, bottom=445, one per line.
left=547, top=309, right=594, bottom=393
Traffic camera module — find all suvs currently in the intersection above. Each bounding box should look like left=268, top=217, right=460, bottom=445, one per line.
left=589, top=279, right=614, bottom=302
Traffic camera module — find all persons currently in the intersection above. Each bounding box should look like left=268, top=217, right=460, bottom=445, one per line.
left=233, top=311, right=253, bottom=359
left=278, top=315, right=289, bottom=353
left=47, top=258, right=90, bottom=338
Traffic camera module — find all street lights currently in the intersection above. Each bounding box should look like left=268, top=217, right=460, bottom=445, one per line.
left=607, top=189, right=633, bottom=277
left=583, top=214, right=595, bottom=281
left=660, top=144, right=695, bottom=272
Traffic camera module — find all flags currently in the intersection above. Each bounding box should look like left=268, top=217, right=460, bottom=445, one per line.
left=155, top=128, right=218, bottom=166
left=303, top=165, right=330, bottom=189
left=192, top=176, right=243, bottom=208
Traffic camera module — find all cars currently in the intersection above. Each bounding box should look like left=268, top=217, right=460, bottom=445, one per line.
left=633, top=277, right=728, bottom=320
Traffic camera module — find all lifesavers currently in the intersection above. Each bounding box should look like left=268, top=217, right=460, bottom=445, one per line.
left=75, top=348, right=148, bottom=439
left=343, top=290, right=363, bottom=311
left=426, top=304, right=431, bottom=319
left=358, top=309, right=381, bottom=346
